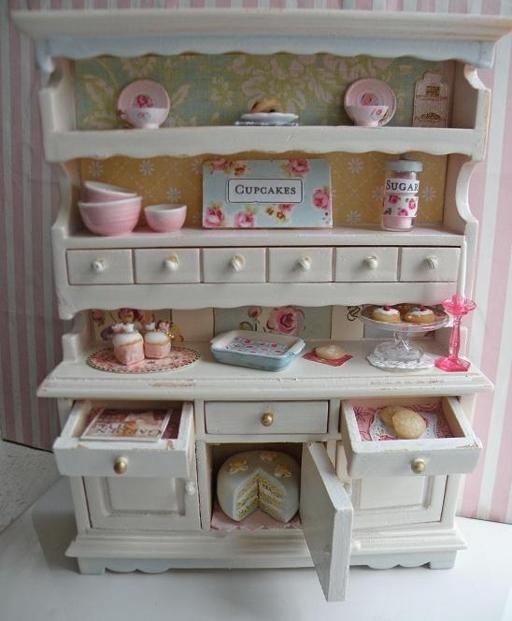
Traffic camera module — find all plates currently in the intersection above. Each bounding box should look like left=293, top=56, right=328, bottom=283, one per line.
left=239, top=112, right=299, bottom=124
left=115, top=81, right=169, bottom=127
left=345, top=78, right=398, bottom=125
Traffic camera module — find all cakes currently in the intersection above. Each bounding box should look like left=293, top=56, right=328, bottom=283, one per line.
left=403, top=305, right=435, bottom=324
left=217, top=450, right=301, bottom=523
left=373, top=305, right=400, bottom=321
left=142, top=320, right=173, bottom=359
left=110, top=322, right=144, bottom=364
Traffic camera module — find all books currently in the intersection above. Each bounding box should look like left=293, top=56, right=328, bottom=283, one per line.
left=80, top=408, right=173, bottom=443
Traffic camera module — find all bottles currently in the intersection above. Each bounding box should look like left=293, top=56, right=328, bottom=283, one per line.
left=379, top=151, right=425, bottom=231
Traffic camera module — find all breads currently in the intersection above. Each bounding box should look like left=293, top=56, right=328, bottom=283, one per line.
left=380, top=407, right=406, bottom=425
left=252, top=99, right=282, bottom=112
left=315, top=344, right=344, bottom=360
left=392, top=411, right=426, bottom=438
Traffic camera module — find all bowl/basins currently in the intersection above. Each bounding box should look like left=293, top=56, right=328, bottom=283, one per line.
left=346, top=104, right=388, bottom=128
left=142, top=203, right=188, bottom=232
left=78, top=180, right=142, bottom=235
left=125, top=108, right=169, bottom=129
